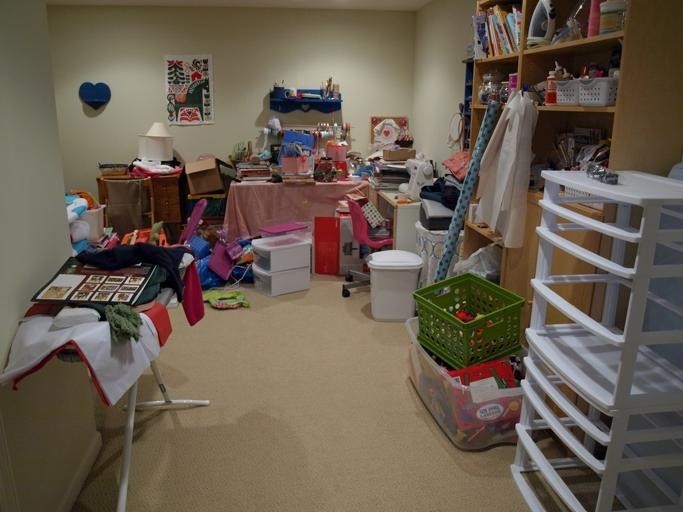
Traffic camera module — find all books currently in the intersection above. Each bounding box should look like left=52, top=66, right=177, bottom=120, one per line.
left=469, top=5, right=521, bottom=64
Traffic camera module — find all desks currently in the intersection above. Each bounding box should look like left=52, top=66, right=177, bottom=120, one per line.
left=96, top=167, right=369, bottom=244
left=19, top=251, right=209, bottom=512
left=369, top=187, right=423, bottom=254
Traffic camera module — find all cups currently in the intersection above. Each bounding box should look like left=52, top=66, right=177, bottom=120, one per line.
left=273, top=87, right=289, bottom=99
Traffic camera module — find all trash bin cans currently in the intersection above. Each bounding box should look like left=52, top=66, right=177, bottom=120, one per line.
left=365, top=249, right=424, bottom=321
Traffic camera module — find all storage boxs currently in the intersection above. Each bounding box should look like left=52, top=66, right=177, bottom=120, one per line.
left=184, top=154, right=233, bottom=195
left=404, top=314, right=527, bottom=452
left=252, top=235, right=312, bottom=299
left=80, top=205, right=108, bottom=241
left=382, top=147, right=416, bottom=160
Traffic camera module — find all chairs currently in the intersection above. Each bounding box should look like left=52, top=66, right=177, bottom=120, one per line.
left=340, top=195, right=394, bottom=297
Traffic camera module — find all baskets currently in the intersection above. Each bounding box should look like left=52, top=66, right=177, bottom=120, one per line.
left=555, top=79, right=578, bottom=104
left=578, top=77, right=619, bottom=106
left=564, top=186, right=604, bottom=210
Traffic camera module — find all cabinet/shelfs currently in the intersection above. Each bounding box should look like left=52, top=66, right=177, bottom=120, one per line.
left=509, top=171, right=683, bottom=510
left=460, top=14, right=683, bottom=421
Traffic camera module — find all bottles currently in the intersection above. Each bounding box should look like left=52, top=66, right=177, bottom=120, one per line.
left=544, top=71, right=558, bottom=107
